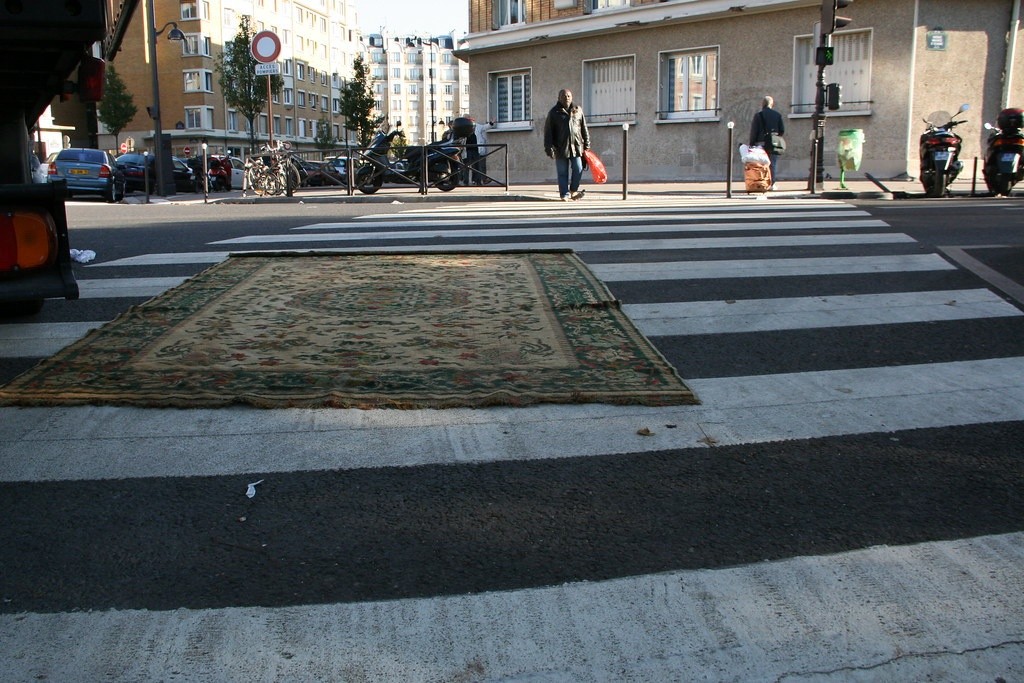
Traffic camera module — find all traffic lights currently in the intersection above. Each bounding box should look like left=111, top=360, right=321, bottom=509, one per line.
left=815, top=46, right=834, bottom=65
left=827, top=82, right=843, bottom=110
left=821, top=0, right=856, bottom=35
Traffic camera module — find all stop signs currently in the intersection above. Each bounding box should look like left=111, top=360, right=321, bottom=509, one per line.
left=183, top=147, right=191, bottom=156
left=121, top=143, right=127, bottom=154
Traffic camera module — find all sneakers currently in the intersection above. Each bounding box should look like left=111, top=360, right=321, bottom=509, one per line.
left=571, top=190, right=585, bottom=200
left=561, top=191, right=571, bottom=202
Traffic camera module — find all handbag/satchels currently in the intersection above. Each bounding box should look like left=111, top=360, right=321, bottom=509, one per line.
left=585, top=150, right=608, bottom=184
left=764, top=131, right=786, bottom=155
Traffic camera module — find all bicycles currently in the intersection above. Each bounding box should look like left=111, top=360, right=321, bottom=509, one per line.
left=241, top=140, right=301, bottom=198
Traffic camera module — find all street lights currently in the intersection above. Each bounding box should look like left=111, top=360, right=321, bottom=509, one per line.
left=417, top=36, right=440, bottom=145
left=142, top=0, right=186, bottom=197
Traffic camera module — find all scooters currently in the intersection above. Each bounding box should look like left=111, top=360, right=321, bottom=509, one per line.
left=981, top=107, right=1024, bottom=197
left=919, top=103, right=970, bottom=199
left=354, top=121, right=463, bottom=195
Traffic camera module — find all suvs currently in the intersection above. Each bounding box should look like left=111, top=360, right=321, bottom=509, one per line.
left=324, top=157, right=362, bottom=184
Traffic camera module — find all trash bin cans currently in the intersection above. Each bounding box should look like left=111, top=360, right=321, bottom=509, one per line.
left=838, top=129, right=866, bottom=172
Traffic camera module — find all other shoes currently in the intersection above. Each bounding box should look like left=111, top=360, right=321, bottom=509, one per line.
left=483, top=178, right=492, bottom=184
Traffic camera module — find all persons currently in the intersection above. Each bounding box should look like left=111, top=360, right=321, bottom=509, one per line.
left=544, top=89, right=590, bottom=202
left=751, top=96, right=785, bottom=191
left=442, top=114, right=497, bottom=186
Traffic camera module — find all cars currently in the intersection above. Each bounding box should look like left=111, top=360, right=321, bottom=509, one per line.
left=46, top=148, right=127, bottom=202
left=208, top=155, right=253, bottom=191
left=113, top=152, right=156, bottom=195
left=306, top=160, right=344, bottom=187
left=172, top=156, right=203, bottom=193
left=0, top=0, right=113, bottom=305
